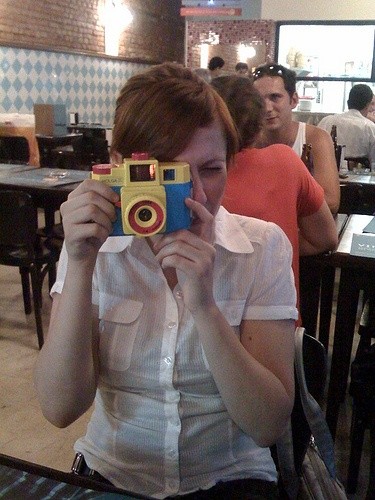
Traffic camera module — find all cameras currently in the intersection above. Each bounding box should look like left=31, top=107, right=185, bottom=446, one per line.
left=91, top=152, right=192, bottom=237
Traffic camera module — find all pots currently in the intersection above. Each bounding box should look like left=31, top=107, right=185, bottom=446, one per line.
left=299, top=101, right=312, bottom=111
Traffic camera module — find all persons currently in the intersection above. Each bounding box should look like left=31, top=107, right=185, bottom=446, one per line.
left=317, top=84, right=375, bottom=170
left=210, top=75, right=338, bottom=326
left=192, top=56, right=250, bottom=82
left=252, top=65, right=341, bottom=215
left=33, top=61, right=298, bottom=500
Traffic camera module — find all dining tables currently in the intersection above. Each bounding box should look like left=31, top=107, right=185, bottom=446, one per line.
left=66, top=125, right=113, bottom=139
left=338, top=174, right=375, bottom=213
left=326, top=214, right=375, bottom=443
left=297, top=214, right=350, bottom=337
left=0, top=164, right=40, bottom=177
left=0, top=164, right=92, bottom=308
left=291, top=106, right=335, bottom=127
left=0, top=453, right=158, bottom=500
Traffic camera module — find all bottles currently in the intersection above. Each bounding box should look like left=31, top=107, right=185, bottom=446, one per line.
left=300, top=143, right=314, bottom=178
left=330, top=125, right=341, bottom=174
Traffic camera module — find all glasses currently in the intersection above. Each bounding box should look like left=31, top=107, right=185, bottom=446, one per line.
left=252, top=66, right=285, bottom=78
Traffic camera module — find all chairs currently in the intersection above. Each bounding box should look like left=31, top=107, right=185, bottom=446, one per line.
left=34, top=150, right=95, bottom=227
left=93, top=134, right=110, bottom=164
left=35, top=224, right=65, bottom=240
left=0, top=135, right=31, bottom=165
left=69, top=333, right=328, bottom=498
left=348, top=297, right=375, bottom=500
left=345, top=157, right=371, bottom=171
left=0, top=190, right=61, bottom=350
left=35, top=133, right=84, bottom=164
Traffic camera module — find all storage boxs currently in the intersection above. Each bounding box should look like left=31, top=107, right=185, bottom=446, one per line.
left=32, top=105, right=67, bottom=137
